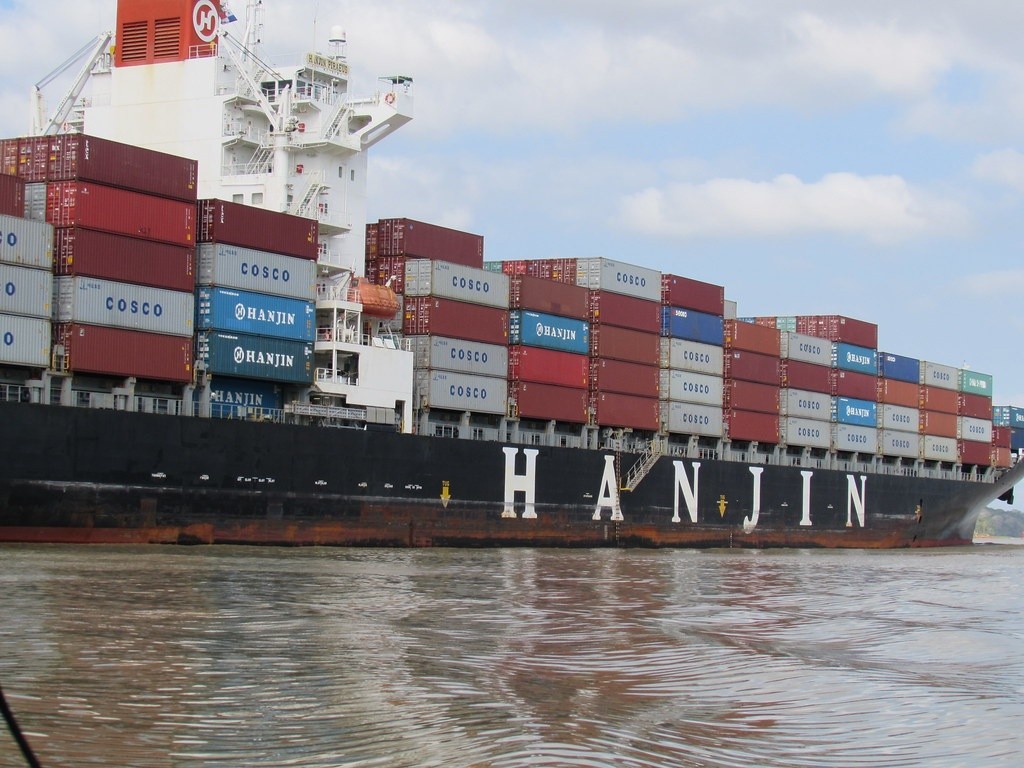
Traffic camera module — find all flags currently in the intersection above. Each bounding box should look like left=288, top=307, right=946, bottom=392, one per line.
left=219, top=4, right=238, bottom=24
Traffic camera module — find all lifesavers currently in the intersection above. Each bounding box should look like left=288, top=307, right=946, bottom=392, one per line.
left=385, top=93, right=395, bottom=104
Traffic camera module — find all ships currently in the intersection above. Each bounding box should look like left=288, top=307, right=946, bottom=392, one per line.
left=0, top=0, right=1024, bottom=552
